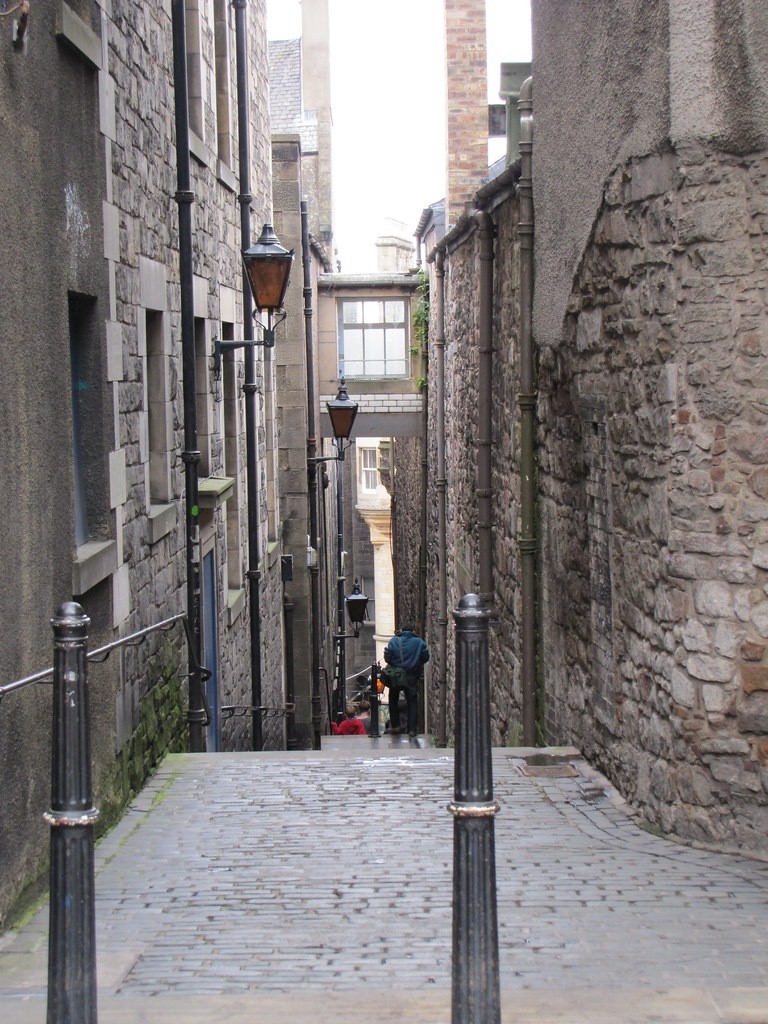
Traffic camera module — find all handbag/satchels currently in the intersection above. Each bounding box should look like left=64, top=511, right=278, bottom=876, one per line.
left=380, top=636, right=407, bottom=690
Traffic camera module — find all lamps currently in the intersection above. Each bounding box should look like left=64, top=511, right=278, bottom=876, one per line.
left=332, top=577, right=369, bottom=642
left=316, top=375, right=359, bottom=462
left=215, top=223, right=295, bottom=370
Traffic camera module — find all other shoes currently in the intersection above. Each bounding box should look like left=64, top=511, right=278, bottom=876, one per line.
left=409, top=731, right=416, bottom=737
left=390, top=726, right=402, bottom=734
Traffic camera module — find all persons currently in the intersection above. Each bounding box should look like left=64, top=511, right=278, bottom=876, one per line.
left=383, top=624, right=430, bottom=737
left=355, top=700, right=371, bottom=734
left=331, top=705, right=367, bottom=735
